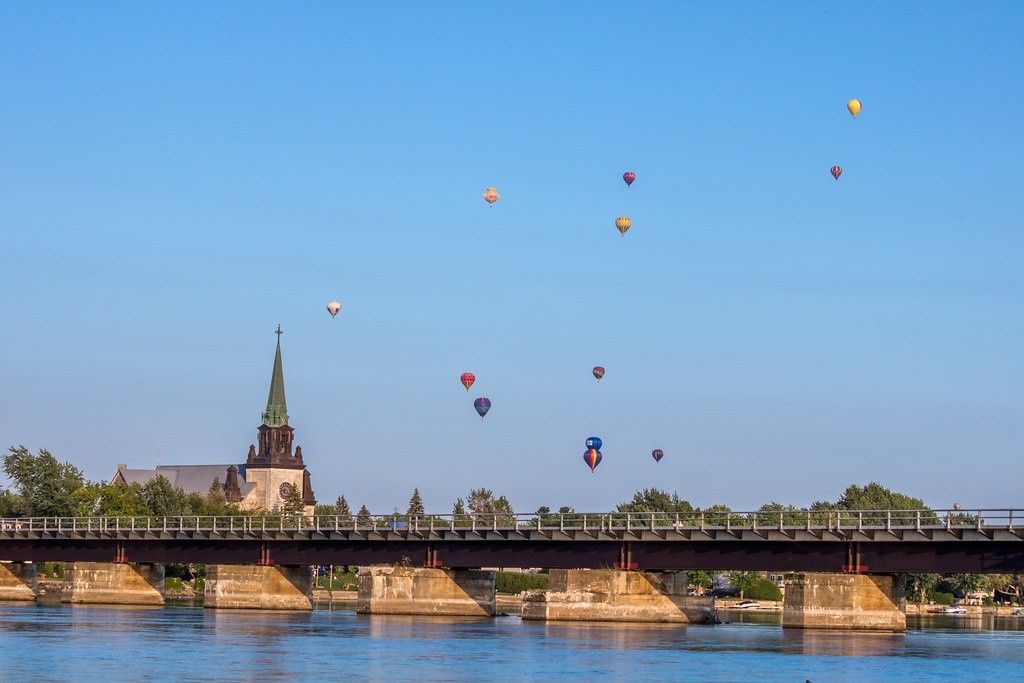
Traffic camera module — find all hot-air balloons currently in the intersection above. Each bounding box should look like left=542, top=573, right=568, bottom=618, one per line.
left=592, top=365, right=606, bottom=385
left=622, top=170, right=635, bottom=189
left=474, top=396, right=492, bottom=421
left=326, top=300, right=344, bottom=321
left=482, top=186, right=500, bottom=210
left=848, top=99, right=863, bottom=121
left=614, top=216, right=632, bottom=239
left=459, top=373, right=476, bottom=394
left=831, top=166, right=843, bottom=184
left=583, top=450, right=603, bottom=475
left=651, top=449, right=664, bottom=466
left=585, top=436, right=604, bottom=450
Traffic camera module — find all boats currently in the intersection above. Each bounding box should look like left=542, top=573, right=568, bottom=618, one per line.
left=938, top=604, right=967, bottom=615
left=729, top=600, right=761, bottom=609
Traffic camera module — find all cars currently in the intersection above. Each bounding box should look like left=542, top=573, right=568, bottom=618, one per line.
left=687, top=587, right=696, bottom=597
